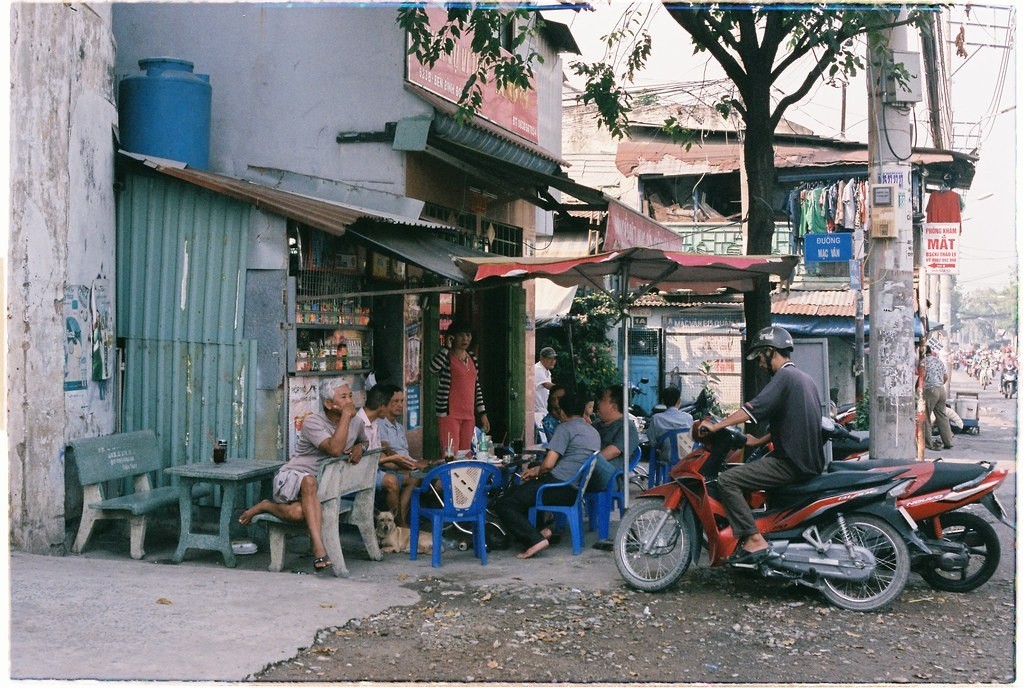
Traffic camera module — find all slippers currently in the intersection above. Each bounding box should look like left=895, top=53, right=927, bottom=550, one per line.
left=313, top=555, right=334, bottom=573
left=722, top=545, right=768, bottom=564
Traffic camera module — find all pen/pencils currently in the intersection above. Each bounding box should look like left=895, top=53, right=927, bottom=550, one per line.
left=447, top=432, right=453, bottom=457
left=515, top=472, right=521, bottom=478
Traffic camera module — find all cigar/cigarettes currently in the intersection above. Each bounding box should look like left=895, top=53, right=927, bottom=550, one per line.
left=515, top=473, right=521, bottom=477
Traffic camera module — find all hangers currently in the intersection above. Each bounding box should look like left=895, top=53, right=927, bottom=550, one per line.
left=938, top=183, right=947, bottom=191
left=798, top=177, right=867, bottom=190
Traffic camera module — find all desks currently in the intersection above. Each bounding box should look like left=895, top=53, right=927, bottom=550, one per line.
left=525, top=444, right=547, bottom=454
left=409, top=454, right=537, bottom=553
left=164, top=457, right=286, bottom=568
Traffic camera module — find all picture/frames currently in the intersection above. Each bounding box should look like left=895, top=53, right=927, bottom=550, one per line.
left=307, top=229, right=360, bottom=273
left=369, top=251, right=407, bottom=284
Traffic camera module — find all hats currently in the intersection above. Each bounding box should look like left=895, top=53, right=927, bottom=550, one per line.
left=540, top=347, right=558, bottom=358
left=746, top=345, right=767, bottom=360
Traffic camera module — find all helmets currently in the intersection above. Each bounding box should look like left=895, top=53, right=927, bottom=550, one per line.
left=748, top=326, right=794, bottom=352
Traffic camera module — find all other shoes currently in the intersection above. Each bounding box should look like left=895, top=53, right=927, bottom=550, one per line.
left=943, top=445, right=953, bottom=449
left=926, top=444, right=934, bottom=450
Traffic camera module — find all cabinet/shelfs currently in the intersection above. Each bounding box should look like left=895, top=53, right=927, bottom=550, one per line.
left=295, top=323, right=374, bottom=377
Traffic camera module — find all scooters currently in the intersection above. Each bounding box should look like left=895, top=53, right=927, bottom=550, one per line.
left=951, top=358, right=1019, bottom=400
left=628, top=378, right=716, bottom=429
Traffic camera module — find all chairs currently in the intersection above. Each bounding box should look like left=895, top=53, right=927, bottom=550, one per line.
left=409, top=446, right=523, bottom=568
left=528, top=446, right=642, bottom=556
left=648, top=428, right=705, bottom=488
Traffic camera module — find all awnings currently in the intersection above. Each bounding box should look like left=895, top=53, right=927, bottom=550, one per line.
left=432, top=134, right=683, bottom=256
left=295, top=227, right=524, bottom=303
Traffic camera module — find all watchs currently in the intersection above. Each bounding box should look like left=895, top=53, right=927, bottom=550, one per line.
left=360, top=443, right=367, bottom=451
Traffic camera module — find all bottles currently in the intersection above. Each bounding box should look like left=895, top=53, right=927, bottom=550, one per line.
left=376, top=256, right=384, bottom=276
left=295, top=300, right=370, bottom=325
left=218, top=440, right=227, bottom=463
left=503, top=448, right=510, bottom=466
left=479, top=428, right=493, bottom=462
left=296, top=336, right=362, bottom=371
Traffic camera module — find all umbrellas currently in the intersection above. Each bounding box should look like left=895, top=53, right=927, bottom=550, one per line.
left=454, top=245, right=772, bottom=514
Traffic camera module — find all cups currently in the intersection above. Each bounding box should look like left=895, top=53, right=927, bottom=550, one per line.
left=445, top=447, right=454, bottom=464
left=214, top=449, right=224, bottom=464
left=494, top=444, right=503, bottom=459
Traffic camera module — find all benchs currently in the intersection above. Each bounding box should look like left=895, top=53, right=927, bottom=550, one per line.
left=69, top=429, right=210, bottom=561
left=251, top=446, right=389, bottom=578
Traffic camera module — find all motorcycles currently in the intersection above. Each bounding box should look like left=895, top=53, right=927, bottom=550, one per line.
left=816, top=403, right=1015, bottom=595
left=613, top=409, right=936, bottom=611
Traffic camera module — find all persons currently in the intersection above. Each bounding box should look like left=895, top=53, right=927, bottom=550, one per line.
left=931, top=403, right=963, bottom=433
left=357, top=384, right=443, bottom=528
left=495, top=393, right=601, bottom=558
left=916, top=345, right=953, bottom=450
left=238, top=380, right=368, bottom=571
left=953, top=345, right=1018, bottom=392
left=697, top=326, right=824, bottom=563
left=830, top=388, right=870, bottom=461
left=542, top=383, right=640, bottom=491
left=429, top=320, right=490, bottom=458
left=647, top=387, right=694, bottom=461
left=535, top=347, right=558, bottom=413
